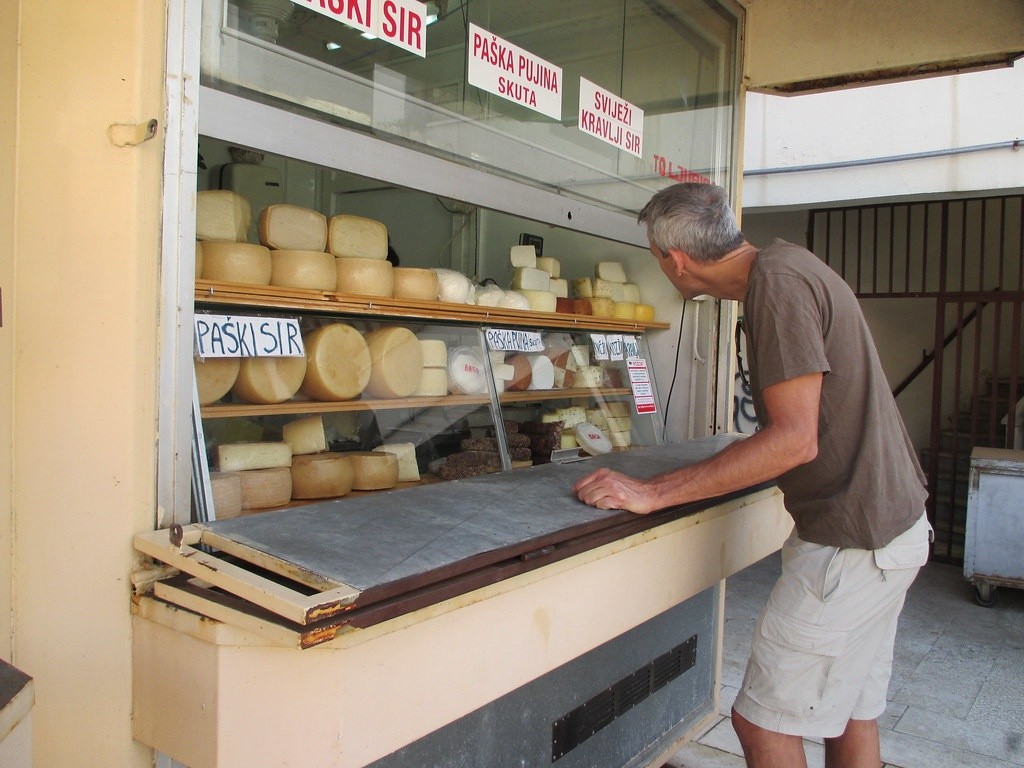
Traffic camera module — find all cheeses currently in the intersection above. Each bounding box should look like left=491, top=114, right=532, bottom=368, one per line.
left=191, top=188, right=658, bottom=520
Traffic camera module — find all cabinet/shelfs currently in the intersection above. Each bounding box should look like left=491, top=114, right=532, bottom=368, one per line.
left=192, top=277, right=670, bottom=525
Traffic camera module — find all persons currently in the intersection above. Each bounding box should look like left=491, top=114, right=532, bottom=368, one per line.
left=354, top=234, right=471, bottom=476
left=573, top=184, right=934, bottom=768
